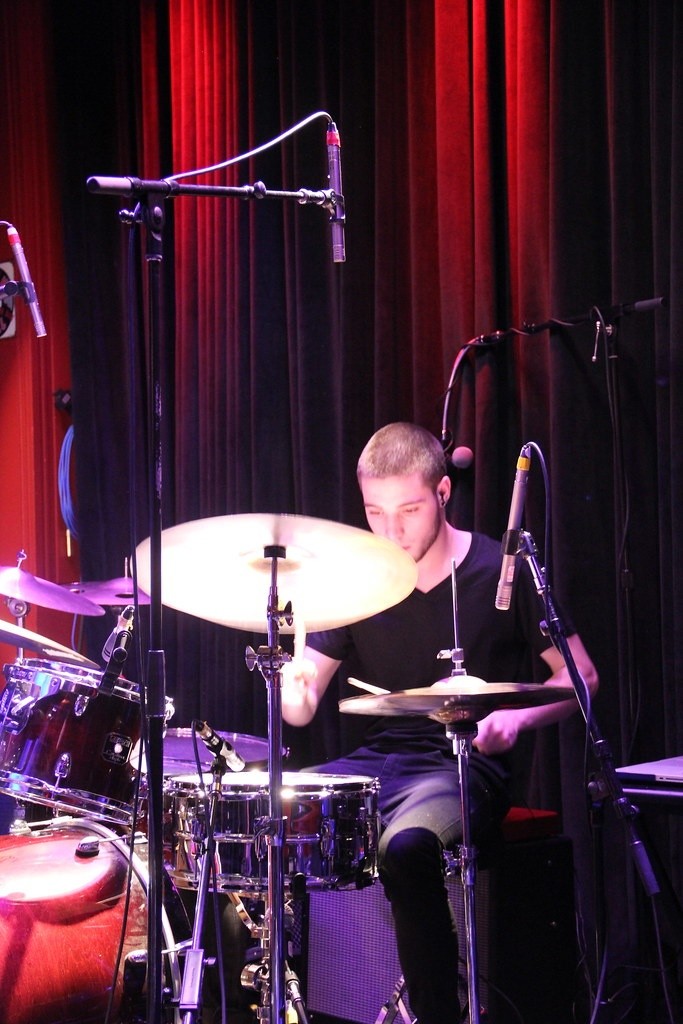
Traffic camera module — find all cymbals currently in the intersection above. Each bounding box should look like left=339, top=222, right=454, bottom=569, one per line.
left=0, top=619, right=101, bottom=669
left=614, top=756, right=683, bottom=779
left=130, top=513, right=419, bottom=635
left=339, top=675, right=576, bottom=724
left=0, top=566, right=106, bottom=616
left=59, top=577, right=150, bottom=605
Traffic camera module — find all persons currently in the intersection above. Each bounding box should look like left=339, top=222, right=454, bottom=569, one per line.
left=280, top=422, right=598, bottom=1023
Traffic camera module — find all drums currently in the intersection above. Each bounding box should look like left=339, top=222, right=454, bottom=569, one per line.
left=0, top=657, right=175, bottom=825
left=0, top=819, right=183, bottom=1024
left=163, top=771, right=381, bottom=893
left=162, top=728, right=289, bottom=778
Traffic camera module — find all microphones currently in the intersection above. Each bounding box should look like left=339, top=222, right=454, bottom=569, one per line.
left=326, top=121, right=347, bottom=264
left=493, top=444, right=532, bottom=612
left=6, top=227, right=47, bottom=340
left=439, top=441, right=474, bottom=471
left=194, top=720, right=246, bottom=774
left=101, top=604, right=134, bottom=663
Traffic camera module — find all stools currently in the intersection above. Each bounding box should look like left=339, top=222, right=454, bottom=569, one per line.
left=306, top=805, right=560, bottom=1024
